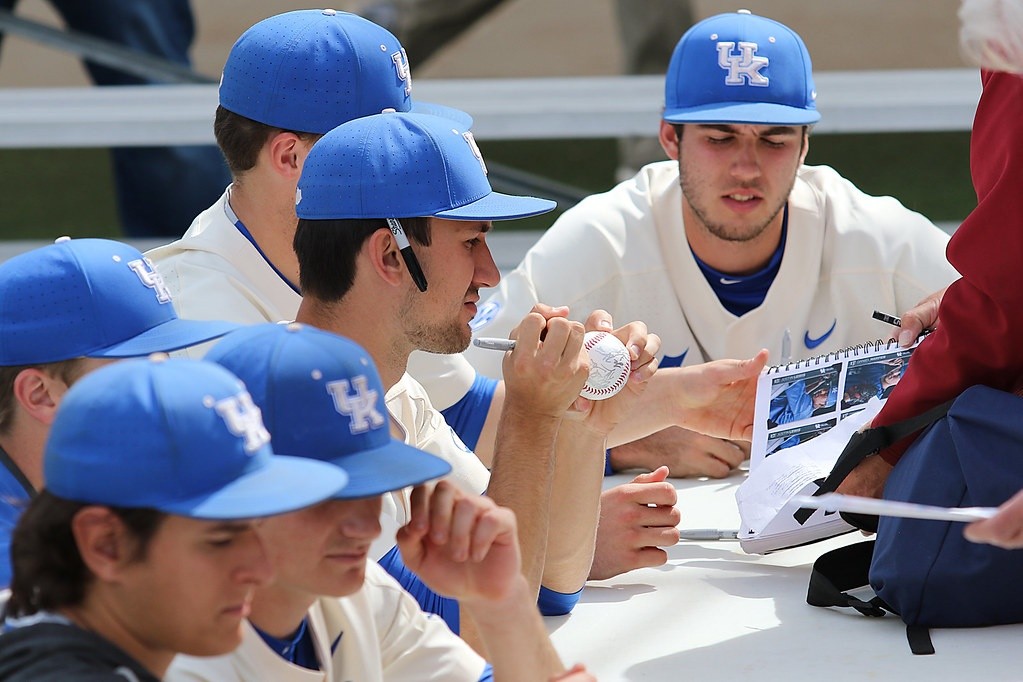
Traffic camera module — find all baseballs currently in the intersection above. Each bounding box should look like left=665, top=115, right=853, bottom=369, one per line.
left=574, top=331, right=631, bottom=400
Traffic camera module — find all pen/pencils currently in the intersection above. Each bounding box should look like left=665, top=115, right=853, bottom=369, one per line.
left=871, top=310, right=932, bottom=335
left=678, top=528, right=737, bottom=540
left=386, top=218, right=428, bottom=293
left=474, top=338, right=518, bottom=352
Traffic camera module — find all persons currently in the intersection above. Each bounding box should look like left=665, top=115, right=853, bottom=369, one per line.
left=464, top=10, right=962, bottom=477
left=843, top=359, right=910, bottom=408
left=769, top=373, right=837, bottom=426
left=834, top=0, right=1023, bottom=549
left=1, top=0, right=773, bottom=682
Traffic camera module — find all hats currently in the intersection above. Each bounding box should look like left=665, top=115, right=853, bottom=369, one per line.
left=200, top=322, right=451, bottom=500
left=295, top=108, right=558, bottom=221
left=0, top=236, right=242, bottom=366
left=42, top=353, right=350, bottom=519
left=824, top=380, right=838, bottom=408
left=899, top=365, right=908, bottom=377
left=662, top=8, right=822, bottom=126
left=218, top=8, right=473, bottom=134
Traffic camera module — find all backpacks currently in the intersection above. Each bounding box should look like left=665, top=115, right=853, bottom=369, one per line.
left=793, top=384, right=1023, bottom=655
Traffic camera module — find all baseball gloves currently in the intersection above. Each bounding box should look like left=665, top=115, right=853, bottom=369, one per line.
left=847, top=384, right=877, bottom=403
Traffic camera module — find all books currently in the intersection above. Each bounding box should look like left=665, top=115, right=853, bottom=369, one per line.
left=736, top=329, right=936, bottom=557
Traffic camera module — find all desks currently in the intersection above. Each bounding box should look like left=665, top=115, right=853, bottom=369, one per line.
left=492, top=455, right=1023, bottom=682
